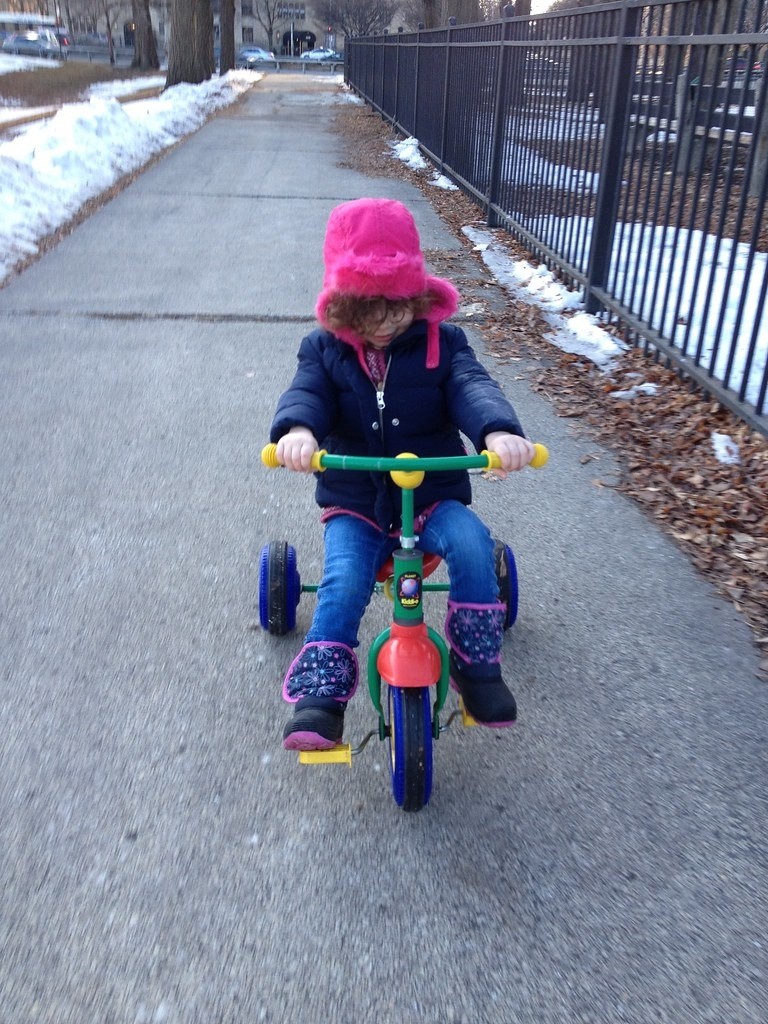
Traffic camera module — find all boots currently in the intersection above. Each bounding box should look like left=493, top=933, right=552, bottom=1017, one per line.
left=445, top=601, right=518, bottom=727
left=282, top=641, right=359, bottom=751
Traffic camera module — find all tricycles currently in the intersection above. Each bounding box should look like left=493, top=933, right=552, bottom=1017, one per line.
left=257, top=444, right=548, bottom=814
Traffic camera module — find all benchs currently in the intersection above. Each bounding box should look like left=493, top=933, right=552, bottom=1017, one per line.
left=599, top=73, right=768, bottom=198
left=252, top=55, right=344, bottom=75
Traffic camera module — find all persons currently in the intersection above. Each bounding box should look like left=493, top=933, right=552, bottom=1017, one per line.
left=263, top=197, right=537, bottom=751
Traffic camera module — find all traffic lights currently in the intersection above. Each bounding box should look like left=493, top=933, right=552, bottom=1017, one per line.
left=328, top=26, right=331, bottom=31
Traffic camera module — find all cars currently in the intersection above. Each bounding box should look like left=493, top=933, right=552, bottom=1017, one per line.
left=239, top=46, right=275, bottom=66
left=319, top=52, right=345, bottom=67
left=4, top=35, right=67, bottom=62
left=301, top=47, right=336, bottom=60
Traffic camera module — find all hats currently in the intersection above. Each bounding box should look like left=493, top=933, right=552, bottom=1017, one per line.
left=318, top=198, right=459, bottom=376
